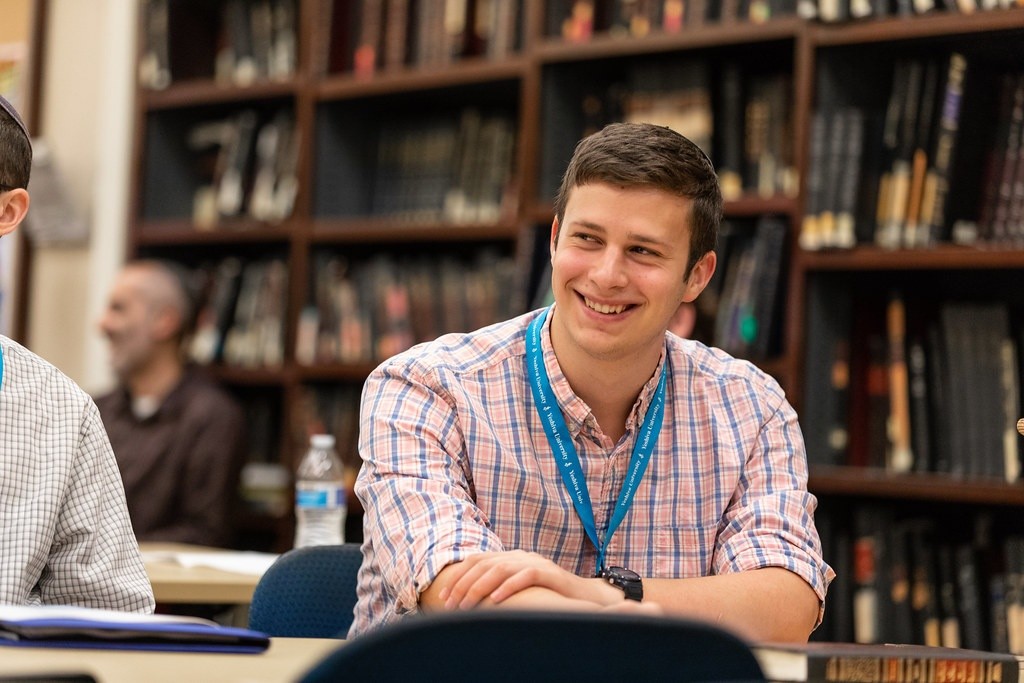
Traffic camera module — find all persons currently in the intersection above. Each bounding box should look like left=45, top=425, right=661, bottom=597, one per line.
left=0, top=92, right=158, bottom=616
left=346, top=122, right=839, bottom=650
left=89, top=263, right=237, bottom=550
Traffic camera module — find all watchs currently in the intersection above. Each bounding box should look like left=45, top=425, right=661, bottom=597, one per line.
left=594, top=565, right=644, bottom=601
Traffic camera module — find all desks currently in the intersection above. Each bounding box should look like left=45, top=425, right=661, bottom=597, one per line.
left=0, top=543, right=807, bottom=683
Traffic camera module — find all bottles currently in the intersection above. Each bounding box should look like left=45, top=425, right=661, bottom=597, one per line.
left=293, top=434, right=348, bottom=550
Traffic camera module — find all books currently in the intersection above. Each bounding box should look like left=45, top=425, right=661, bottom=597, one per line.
left=127, top=0, right=1024, bottom=656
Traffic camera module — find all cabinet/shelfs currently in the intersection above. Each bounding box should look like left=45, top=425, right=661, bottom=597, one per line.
left=122, top=0, right=1024, bottom=506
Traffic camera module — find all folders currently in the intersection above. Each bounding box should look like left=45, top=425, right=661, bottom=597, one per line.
left=0, top=617, right=271, bottom=656
